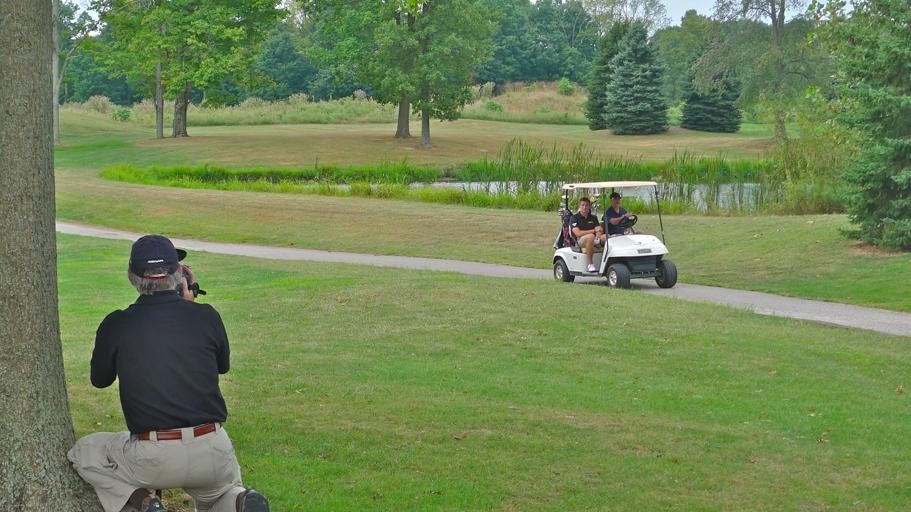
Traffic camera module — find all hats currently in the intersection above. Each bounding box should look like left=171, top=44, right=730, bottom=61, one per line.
left=610, top=193, right=622, bottom=199
left=129, top=234, right=186, bottom=279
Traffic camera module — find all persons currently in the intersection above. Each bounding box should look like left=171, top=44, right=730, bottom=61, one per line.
left=604, top=193, right=632, bottom=237
left=569, top=197, right=610, bottom=271
left=66, top=236, right=269, bottom=512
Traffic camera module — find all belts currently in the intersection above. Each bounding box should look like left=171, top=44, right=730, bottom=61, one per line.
left=137, top=422, right=224, bottom=442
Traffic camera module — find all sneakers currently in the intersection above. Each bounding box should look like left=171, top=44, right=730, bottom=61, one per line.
left=140, top=496, right=165, bottom=511
left=235, top=488, right=270, bottom=512
left=588, top=264, right=597, bottom=272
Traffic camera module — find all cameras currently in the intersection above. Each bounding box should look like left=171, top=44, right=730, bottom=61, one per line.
left=175, top=266, right=191, bottom=292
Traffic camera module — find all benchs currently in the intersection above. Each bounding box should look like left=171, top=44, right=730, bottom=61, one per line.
left=568, top=221, right=604, bottom=254
